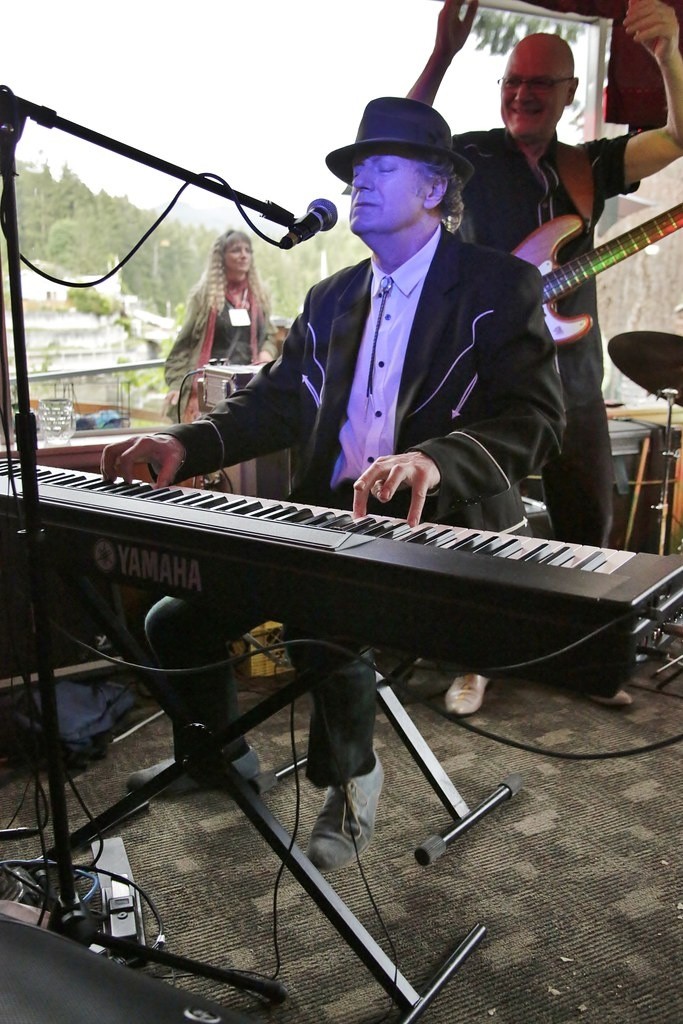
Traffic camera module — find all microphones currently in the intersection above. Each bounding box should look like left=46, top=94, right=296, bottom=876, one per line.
left=280, top=199, right=337, bottom=249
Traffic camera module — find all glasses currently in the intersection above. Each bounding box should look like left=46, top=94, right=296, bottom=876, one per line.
left=498, top=75, right=575, bottom=93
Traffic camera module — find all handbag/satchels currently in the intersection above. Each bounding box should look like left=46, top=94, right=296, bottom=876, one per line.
left=9, top=678, right=135, bottom=749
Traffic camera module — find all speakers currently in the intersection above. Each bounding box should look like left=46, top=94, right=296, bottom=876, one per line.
left=0, top=541, right=128, bottom=691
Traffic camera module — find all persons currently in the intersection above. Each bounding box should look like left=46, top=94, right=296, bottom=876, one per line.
left=100, top=97, right=565, bottom=870
left=402, top=0, right=683, bottom=716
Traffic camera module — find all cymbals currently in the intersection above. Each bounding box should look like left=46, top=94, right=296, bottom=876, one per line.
left=608, top=331, right=683, bottom=407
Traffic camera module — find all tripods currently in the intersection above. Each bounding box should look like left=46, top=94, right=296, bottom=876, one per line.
left=0, top=83, right=296, bottom=1007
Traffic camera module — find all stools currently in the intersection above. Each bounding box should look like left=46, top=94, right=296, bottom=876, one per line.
left=247, top=649, right=517, bottom=865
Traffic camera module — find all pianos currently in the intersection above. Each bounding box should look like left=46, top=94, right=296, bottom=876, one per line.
left=1, top=456, right=682, bottom=651
left=201, top=362, right=264, bottom=410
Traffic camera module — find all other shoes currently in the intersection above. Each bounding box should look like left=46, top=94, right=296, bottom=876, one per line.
left=127, top=743, right=261, bottom=792
left=305, top=749, right=384, bottom=874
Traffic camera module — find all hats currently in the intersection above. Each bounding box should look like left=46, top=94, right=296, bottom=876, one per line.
left=325, top=96, right=475, bottom=187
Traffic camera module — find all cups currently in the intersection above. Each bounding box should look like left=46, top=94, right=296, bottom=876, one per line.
left=38, top=398, right=76, bottom=446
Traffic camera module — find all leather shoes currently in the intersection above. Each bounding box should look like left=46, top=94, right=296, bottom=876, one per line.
left=444, top=670, right=489, bottom=715
left=584, top=688, right=633, bottom=707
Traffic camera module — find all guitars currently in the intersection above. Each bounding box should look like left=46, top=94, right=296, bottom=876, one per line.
left=510, top=202, right=683, bottom=349
